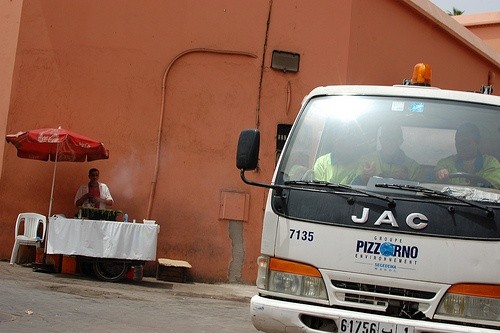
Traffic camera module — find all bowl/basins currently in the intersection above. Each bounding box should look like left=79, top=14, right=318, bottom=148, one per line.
left=143, top=219, right=156, bottom=224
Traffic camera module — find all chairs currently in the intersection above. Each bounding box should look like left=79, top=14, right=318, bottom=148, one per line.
left=10, top=212, right=47, bottom=268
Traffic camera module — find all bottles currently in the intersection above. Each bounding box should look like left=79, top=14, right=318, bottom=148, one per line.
left=75, top=207, right=117, bottom=221
left=124, top=211, right=129, bottom=222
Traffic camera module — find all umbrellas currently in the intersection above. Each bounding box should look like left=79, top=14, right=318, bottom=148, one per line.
left=5, top=125, right=110, bottom=264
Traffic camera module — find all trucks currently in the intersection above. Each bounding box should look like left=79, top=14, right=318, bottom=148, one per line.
left=236, top=62, right=500, bottom=333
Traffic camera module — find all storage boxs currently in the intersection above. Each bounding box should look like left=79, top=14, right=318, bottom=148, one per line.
left=156, top=263, right=187, bottom=282
left=36, top=246, right=61, bottom=273
left=62, top=254, right=76, bottom=275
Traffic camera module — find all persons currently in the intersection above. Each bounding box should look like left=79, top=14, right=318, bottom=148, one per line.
left=74, top=168, right=115, bottom=278
left=313, top=120, right=500, bottom=191
